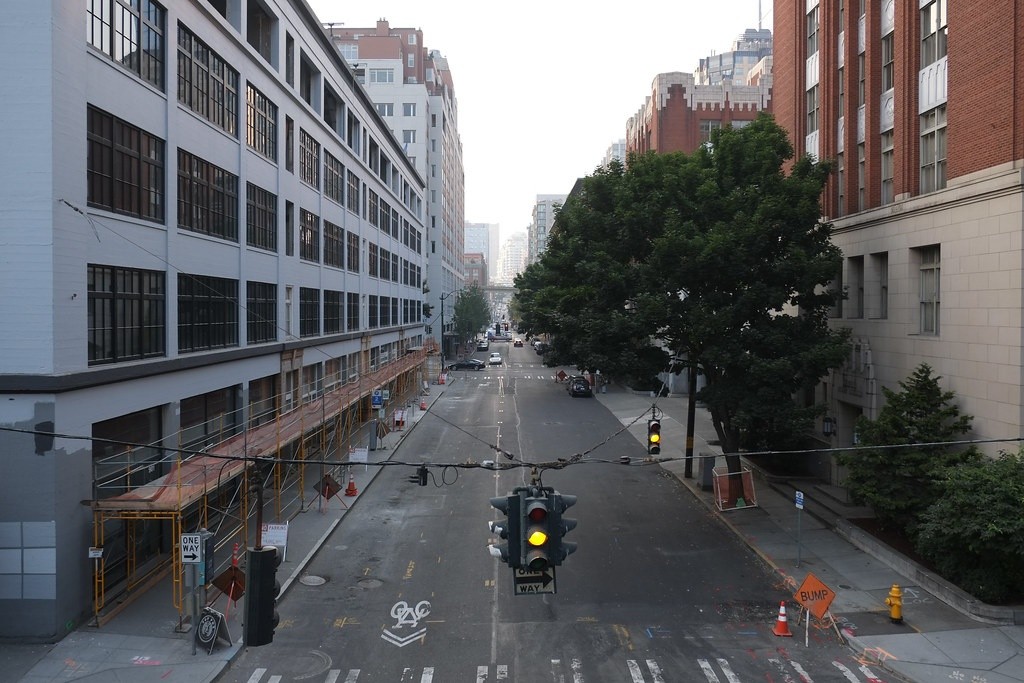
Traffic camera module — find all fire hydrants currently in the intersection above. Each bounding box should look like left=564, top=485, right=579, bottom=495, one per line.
left=884, top=584, right=904, bottom=624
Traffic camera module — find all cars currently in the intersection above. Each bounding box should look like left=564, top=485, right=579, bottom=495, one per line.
left=565, top=375, right=591, bottom=398
left=477, top=340, right=489, bottom=351
left=487, top=320, right=550, bottom=355
left=448, top=359, right=485, bottom=371
left=488, top=352, right=502, bottom=365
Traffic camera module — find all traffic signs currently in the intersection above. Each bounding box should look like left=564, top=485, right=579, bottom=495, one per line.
left=513, top=568, right=557, bottom=595
left=180, top=534, right=201, bottom=565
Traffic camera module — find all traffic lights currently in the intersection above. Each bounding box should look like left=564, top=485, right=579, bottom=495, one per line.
left=487, top=494, right=520, bottom=568
left=648, top=419, right=660, bottom=454
left=555, top=493, right=578, bottom=566
left=523, top=497, right=552, bottom=571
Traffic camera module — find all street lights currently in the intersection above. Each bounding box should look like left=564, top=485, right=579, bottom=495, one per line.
left=441, top=289, right=470, bottom=372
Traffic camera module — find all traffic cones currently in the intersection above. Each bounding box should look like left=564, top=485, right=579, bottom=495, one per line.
left=771, top=601, right=793, bottom=636
left=420, top=397, right=427, bottom=411
left=437, top=374, right=442, bottom=385
left=345, top=473, right=358, bottom=496
